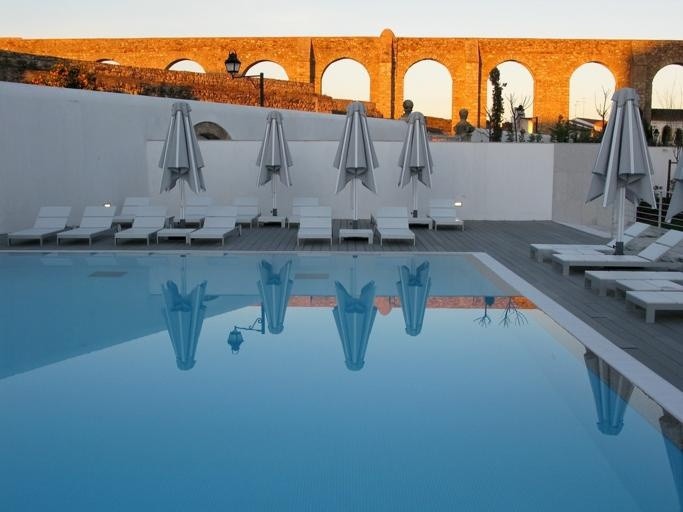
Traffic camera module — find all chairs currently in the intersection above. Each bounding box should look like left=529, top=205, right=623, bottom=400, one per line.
left=116, top=195, right=238, bottom=250
left=8, top=204, right=72, bottom=248
left=56, top=205, right=116, bottom=248
left=368, top=206, right=418, bottom=246
left=424, top=198, right=464, bottom=231
left=530, top=221, right=683, bottom=324
left=234, top=196, right=260, bottom=230
left=286, top=195, right=334, bottom=244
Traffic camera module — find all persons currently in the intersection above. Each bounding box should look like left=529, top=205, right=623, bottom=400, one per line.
left=453, top=108, right=475, bottom=135
left=395, top=99, right=413, bottom=121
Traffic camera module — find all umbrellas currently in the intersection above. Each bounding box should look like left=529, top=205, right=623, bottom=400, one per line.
left=157, top=101, right=206, bottom=228
left=584, top=86, right=658, bottom=255
left=254, top=111, right=293, bottom=215
left=665, top=147, right=683, bottom=224
left=332, top=101, right=379, bottom=228
left=658, top=408, right=683, bottom=512
left=397, top=114, right=434, bottom=218
left=155, top=256, right=432, bottom=371
left=582, top=346, right=634, bottom=436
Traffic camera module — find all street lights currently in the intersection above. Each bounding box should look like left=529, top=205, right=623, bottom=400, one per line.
left=223, top=49, right=264, bottom=106
left=516, top=104, right=539, bottom=132
left=227, top=302, right=266, bottom=355
